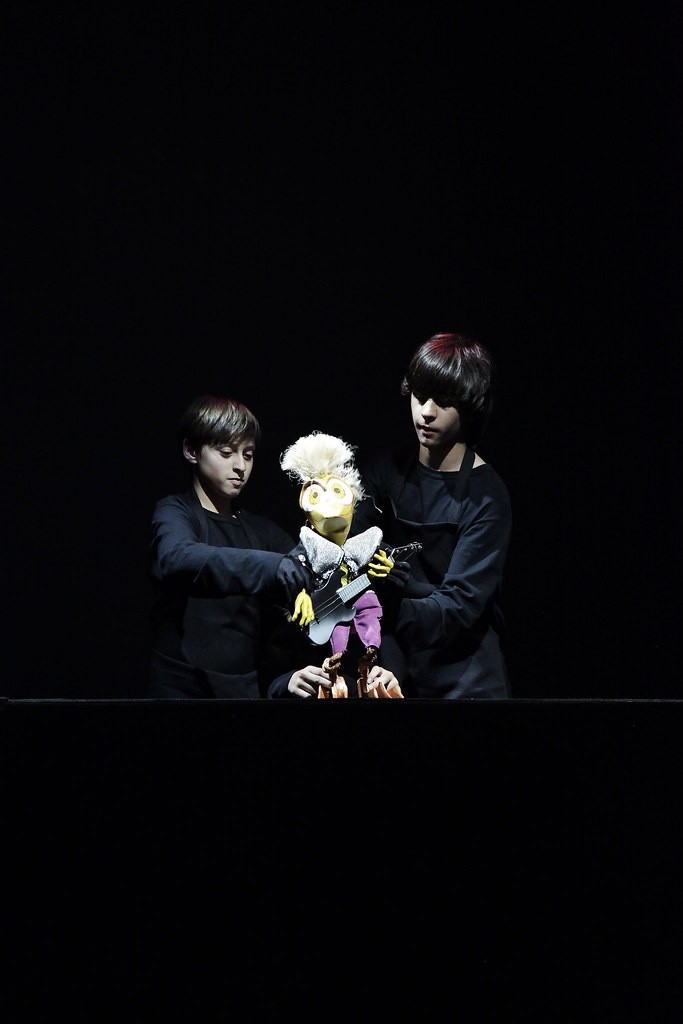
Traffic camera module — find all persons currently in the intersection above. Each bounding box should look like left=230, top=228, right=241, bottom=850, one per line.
left=267, top=662, right=407, bottom=700
left=142, top=396, right=417, bottom=700
left=347, top=332, right=512, bottom=700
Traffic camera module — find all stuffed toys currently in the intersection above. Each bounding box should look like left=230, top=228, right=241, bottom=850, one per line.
left=280, top=429, right=401, bottom=698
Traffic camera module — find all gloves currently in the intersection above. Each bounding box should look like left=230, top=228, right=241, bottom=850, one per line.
left=275, top=555, right=315, bottom=603
left=377, top=559, right=411, bottom=606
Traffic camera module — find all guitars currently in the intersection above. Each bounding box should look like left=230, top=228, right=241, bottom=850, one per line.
left=297, top=541, right=424, bottom=648
